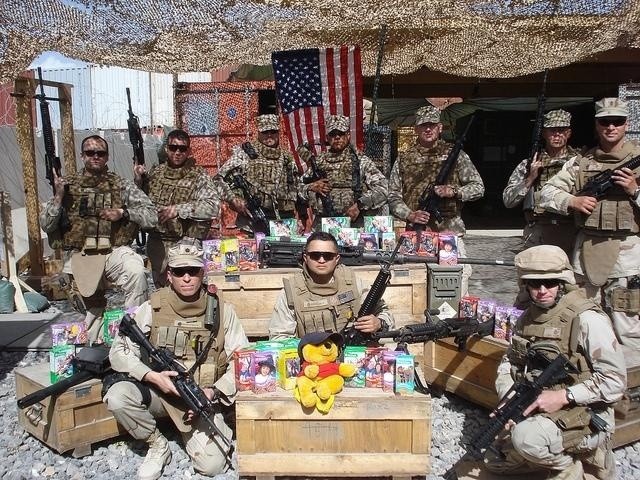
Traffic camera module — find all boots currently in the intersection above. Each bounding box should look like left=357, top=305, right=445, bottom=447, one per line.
left=136, top=428, right=172, bottom=480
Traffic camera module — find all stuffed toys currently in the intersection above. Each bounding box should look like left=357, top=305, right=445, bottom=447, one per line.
left=291, top=332, right=356, bottom=416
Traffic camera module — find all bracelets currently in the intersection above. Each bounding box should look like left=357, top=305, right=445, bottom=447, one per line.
left=356, top=200, right=363, bottom=210
left=451, top=186, right=458, bottom=199
left=209, top=386, right=220, bottom=401
left=630, top=187, right=640, bottom=200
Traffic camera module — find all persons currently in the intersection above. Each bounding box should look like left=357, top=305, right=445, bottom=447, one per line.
left=214, top=112, right=301, bottom=234
left=254, top=359, right=277, bottom=392
left=132, top=130, right=224, bottom=289
left=540, top=98, right=640, bottom=372
left=296, top=113, right=388, bottom=230
left=37, top=134, right=160, bottom=311
left=268, top=231, right=396, bottom=347
left=101, top=242, right=250, bottom=480
left=388, top=104, right=485, bottom=299
left=502, top=109, right=589, bottom=310
left=383, top=360, right=396, bottom=383
left=438, top=238, right=458, bottom=266
left=482, top=244, right=629, bottom=480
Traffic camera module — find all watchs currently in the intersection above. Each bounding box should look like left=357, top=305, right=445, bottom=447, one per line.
left=567, top=388, right=575, bottom=408
left=379, top=319, right=388, bottom=334
left=124, top=207, right=129, bottom=220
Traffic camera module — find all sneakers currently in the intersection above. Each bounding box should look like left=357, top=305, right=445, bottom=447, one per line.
left=544, top=460, right=584, bottom=480
left=578, top=435, right=616, bottom=479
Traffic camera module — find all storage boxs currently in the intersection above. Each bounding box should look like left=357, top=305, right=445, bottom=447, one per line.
left=234, top=387, right=431, bottom=480
left=423, top=336, right=640, bottom=450
left=205, top=261, right=428, bottom=337
left=13, top=362, right=129, bottom=455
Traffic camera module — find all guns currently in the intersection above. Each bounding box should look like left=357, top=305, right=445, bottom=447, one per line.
left=119, top=316, right=235, bottom=448
left=525, top=70, right=554, bottom=186
left=338, top=235, right=406, bottom=348
left=229, top=173, right=273, bottom=236
left=125, top=88, right=149, bottom=192
left=413, top=113, right=476, bottom=233
left=308, top=155, right=335, bottom=217
left=467, top=354, right=608, bottom=461
left=567, top=157, right=640, bottom=232
left=37, top=66, right=63, bottom=196
left=371, top=312, right=495, bottom=351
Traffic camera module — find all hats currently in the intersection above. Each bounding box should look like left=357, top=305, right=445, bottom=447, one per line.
left=513, top=244, right=576, bottom=311
left=414, top=105, right=441, bottom=127
left=325, top=115, right=350, bottom=135
left=257, top=114, right=281, bottom=133
left=594, top=97, right=629, bottom=119
left=543, top=109, right=572, bottom=128
left=167, top=244, right=205, bottom=268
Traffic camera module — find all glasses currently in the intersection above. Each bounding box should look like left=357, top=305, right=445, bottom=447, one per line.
left=167, top=144, right=189, bottom=152
left=598, top=117, right=626, bottom=127
left=527, top=279, right=561, bottom=288
left=82, top=150, right=108, bottom=157
left=328, top=129, right=349, bottom=137
left=258, top=130, right=278, bottom=134
left=171, top=267, right=202, bottom=277
left=307, top=251, right=338, bottom=260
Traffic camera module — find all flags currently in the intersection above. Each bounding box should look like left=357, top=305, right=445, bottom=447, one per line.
left=270, top=45, right=365, bottom=177
